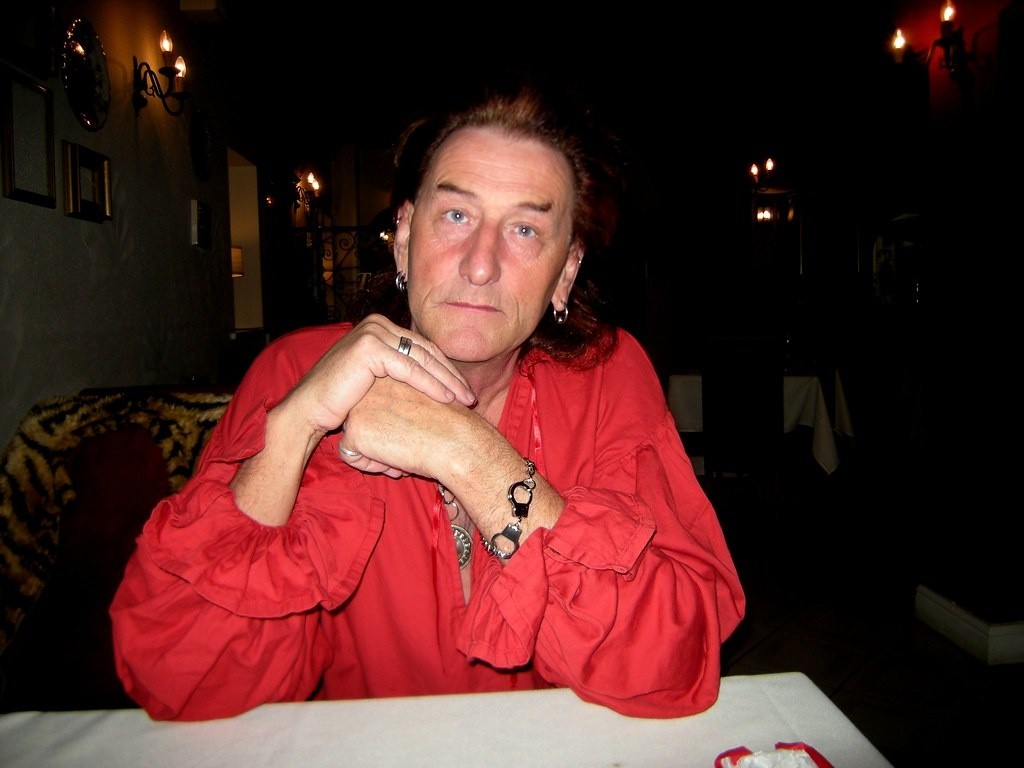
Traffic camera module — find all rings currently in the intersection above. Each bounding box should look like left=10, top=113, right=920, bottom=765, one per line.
left=397, top=336, right=413, bottom=355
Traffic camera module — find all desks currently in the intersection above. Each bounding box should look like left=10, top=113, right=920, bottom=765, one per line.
left=0, top=668, right=893, bottom=768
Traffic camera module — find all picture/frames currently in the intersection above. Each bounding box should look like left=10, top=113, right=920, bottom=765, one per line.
left=190, top=199, right=216, bottom=253
left=0, top=0, right=66, bottom=83
left=0, top=61, right=58, bottom=209
left=61, top=138, right=113, bottom=225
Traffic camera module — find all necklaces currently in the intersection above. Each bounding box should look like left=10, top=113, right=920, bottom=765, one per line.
left=435, top=484, right=473, bottom=569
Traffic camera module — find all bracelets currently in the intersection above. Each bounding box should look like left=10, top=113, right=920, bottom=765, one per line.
left=478, top=457, right=535, bottom=558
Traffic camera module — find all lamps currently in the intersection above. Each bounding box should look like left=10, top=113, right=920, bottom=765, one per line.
left=129, top=27, right=189, bottom=120
left=886, top=0, right=974, bottom=119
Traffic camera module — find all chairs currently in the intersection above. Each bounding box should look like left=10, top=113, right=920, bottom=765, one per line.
left=0, top=385, right=240, bottom=713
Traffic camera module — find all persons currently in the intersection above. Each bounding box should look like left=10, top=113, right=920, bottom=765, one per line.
left=108, top=81, right=746, bottom=721
